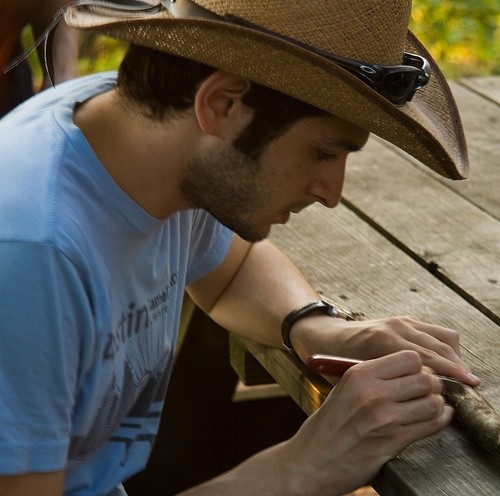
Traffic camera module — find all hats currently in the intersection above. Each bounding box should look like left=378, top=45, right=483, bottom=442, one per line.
left=64, top=1, right=470, bottom=181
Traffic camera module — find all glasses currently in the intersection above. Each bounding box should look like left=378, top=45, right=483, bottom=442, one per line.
left=226, top=12, right=431, bottom=108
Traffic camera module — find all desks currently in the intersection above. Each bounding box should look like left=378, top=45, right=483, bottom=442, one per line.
left=180, top=73, right=500, bottom=496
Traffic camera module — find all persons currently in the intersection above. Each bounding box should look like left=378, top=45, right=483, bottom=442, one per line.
left=0, top=1, right=479, bottom=496
left=0, top=0, right=81, bottom=115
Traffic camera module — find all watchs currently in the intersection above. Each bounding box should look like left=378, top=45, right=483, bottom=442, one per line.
left=282, top=292, right=355, bottom=362
